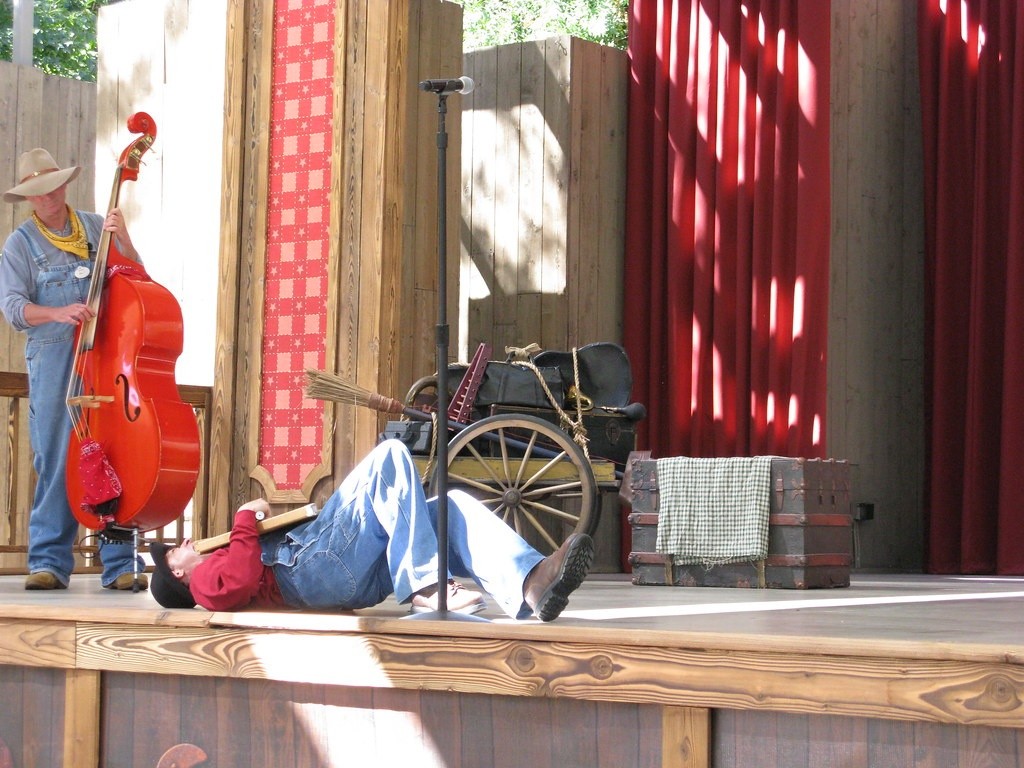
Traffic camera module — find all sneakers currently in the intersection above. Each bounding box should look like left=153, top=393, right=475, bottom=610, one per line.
left=410, top=579, right=488, bottom=615
left=522, top=532, right=595, bottom=623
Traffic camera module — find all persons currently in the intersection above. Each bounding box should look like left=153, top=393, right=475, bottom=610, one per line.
left=0, top=147, right=148, bottom=591
left=150, top=438, right=595, bottom=621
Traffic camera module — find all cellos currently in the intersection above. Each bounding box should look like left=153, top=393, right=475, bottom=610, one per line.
left=62, top=110, right=202, bottom=594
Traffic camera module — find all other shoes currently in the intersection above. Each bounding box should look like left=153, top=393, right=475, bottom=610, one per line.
left=103, top=573, right=149, bottom=590
left=24, top=572, right=68, bottom=589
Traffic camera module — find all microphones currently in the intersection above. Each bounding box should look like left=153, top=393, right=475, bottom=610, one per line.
left=418, top=75, right=475, bottom=95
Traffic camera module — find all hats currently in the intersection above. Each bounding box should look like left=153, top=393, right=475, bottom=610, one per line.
left=150, top=541, right=196, bottom=609
left=3, top=148, right=82, bottom=202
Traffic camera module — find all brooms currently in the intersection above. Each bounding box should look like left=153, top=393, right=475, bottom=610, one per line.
left=305, top=368, right=571, bottom=464
